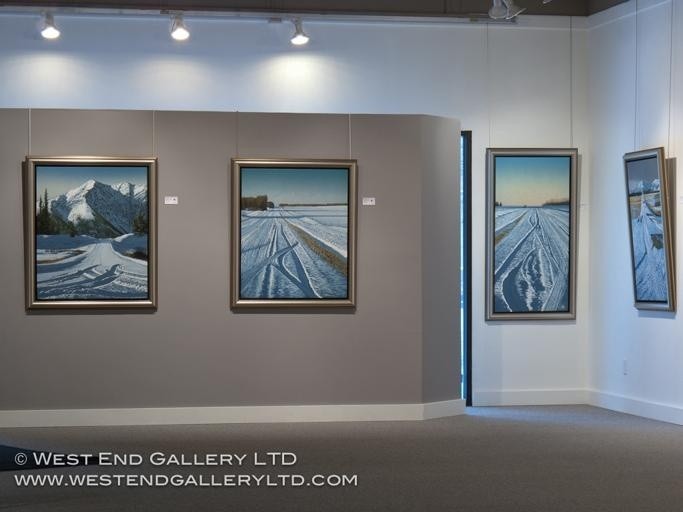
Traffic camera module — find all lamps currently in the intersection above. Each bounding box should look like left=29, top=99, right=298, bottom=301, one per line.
left=488, top=0, right=527, bottom=20
left=39, top=10, right=310, bottom=46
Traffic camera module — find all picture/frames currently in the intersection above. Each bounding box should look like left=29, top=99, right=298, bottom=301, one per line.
left=230, top=158, right=357, bottom=308
left=25, top=155, right=157, bottom=310
left=487, top=148, right=578, bottom=320
left=623, top=147, right=676, bottom=311
